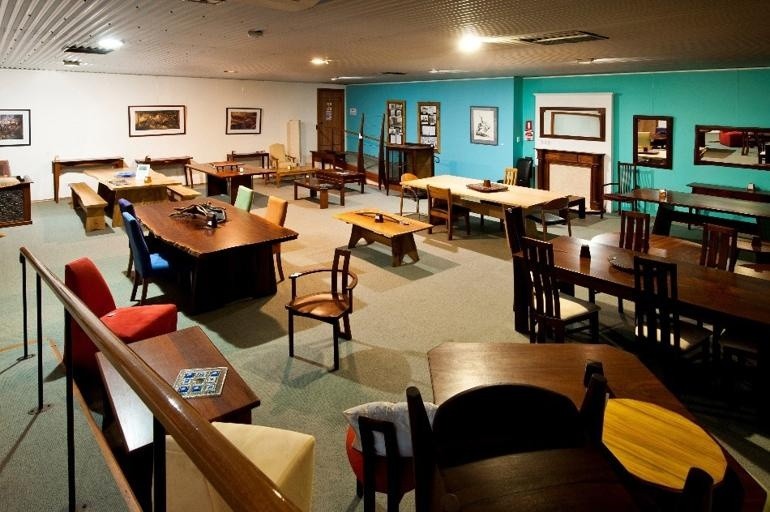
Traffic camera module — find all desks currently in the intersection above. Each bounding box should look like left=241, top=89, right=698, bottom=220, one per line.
left=0, top=175, right=34, bottom=228
left=316, top=168, right=365, bottom=194
left=294, top=177, right=345, bottom=209
left=52, top=157, right=124, bottom=203
left=332, top=208, right=434, bottom=268
left=134, top=156, right=195, bottom=188
left=227, top=153, right=269, bottom=184
left=310, top=150, right=346, bottom=169
left=272, top=167, right=321, bottom=188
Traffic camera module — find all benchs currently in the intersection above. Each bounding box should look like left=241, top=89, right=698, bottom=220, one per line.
left=167, top=186, right=202, bottom=202
left=69, top=182, right=108, bottom=232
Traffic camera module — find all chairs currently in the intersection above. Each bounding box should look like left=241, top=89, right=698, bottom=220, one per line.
left=288, top=249, right=358, bottom=370
left=345, top=401, right=415, bottom=512
left=427, top=183, right=472, bottom=240
left=526, top=194, right=571, bottom=241
left=64, top=257, right=176, bottom=376
left=270, top=144, right=296, bottom=169
left=505, top=167, right=518, bottom=184
left=598, top=161, right=641, bottom=219
left=503, top=206, right=539, bottom=256
left=618, top=210, right=651, bottom=313
left=408, top=358, right=641, bottom=512
left=696, top=222, right=738, bottom=334
left=0, top=159, right=11, bottom=177
left=262, top=196, right=288, bottom=281
left=123, top=212, right=187, bottom=305
left=166, top=421, right=315, bottom=512
left=632, top=255, right=713, bottom=385
left=118, top=199, right=160, bottom=277
left=233, top=185, right=254, bottom=211
left=399, top=173, right=422, bottom=217
left=522, top=236, right=601, bottom=345
left=497, top=158, right=533, bottom=186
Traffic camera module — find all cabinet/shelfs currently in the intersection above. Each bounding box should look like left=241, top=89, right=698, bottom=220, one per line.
left=385, top=144, right=435, bottom=200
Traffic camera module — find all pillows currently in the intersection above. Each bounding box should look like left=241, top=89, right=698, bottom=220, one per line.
left=342, top=401, right=438, bottom=459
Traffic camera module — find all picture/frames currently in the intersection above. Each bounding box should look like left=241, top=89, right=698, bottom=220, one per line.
left=694, top=125, right=770, bottom=171
left=0, top=109, right=31, bottom=147
left=417, top=102, right=440, bottom=154
left=470, top=105, right=498, bottom=145
left=226, top=107, right=261, bottom=134
left=127, top=104, right=186, bottom=137
left=540, top=107, right=606, bottom=142
left=386, top=99, right=406, bottom=145
left=632, top=114, right=674, bottom=170
